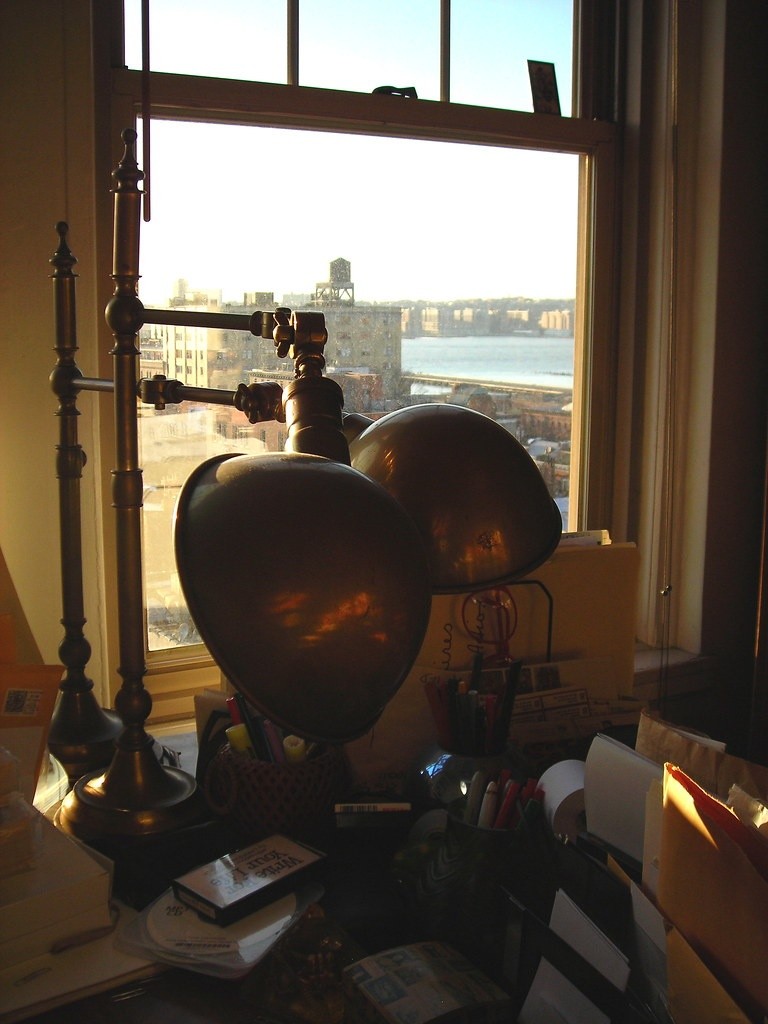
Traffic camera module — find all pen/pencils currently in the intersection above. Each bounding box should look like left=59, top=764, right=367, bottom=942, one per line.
left=422, top=651, right=495, bottom=757
left=460, top=766, right=547, bottom=830
left=224, top=686, right=307, bottom=767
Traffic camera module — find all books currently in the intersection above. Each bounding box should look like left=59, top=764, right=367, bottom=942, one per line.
left=343, top=940, right=512, bottom=1024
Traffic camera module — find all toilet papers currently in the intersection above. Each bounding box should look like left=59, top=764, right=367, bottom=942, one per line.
left=536, top=759, right=585, bottom=834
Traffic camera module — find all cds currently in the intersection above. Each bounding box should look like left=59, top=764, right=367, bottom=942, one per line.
left=145, top=886, right=296, bottom=963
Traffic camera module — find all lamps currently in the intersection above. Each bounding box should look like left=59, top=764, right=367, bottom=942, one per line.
left=56, top=127, right=431, bottom=852
left=45, top=217, right=561, bottom=785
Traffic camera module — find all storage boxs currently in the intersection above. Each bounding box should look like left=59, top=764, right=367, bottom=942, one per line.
left=212, top=738, right=357, bottom=846
left=173, top=834, right=329, bottom=927
left=508, top=684, right=591, bottom=724
left=482, top=663, right=560, bottom=694
left=0, top=549, right=68, bottom=806
left=0, top=791, right=111, bottom=972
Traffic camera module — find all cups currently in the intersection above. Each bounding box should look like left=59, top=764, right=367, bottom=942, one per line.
left=410, top=739, right=522, bottom=871
left=447, top=805, right=539, bottom=918
